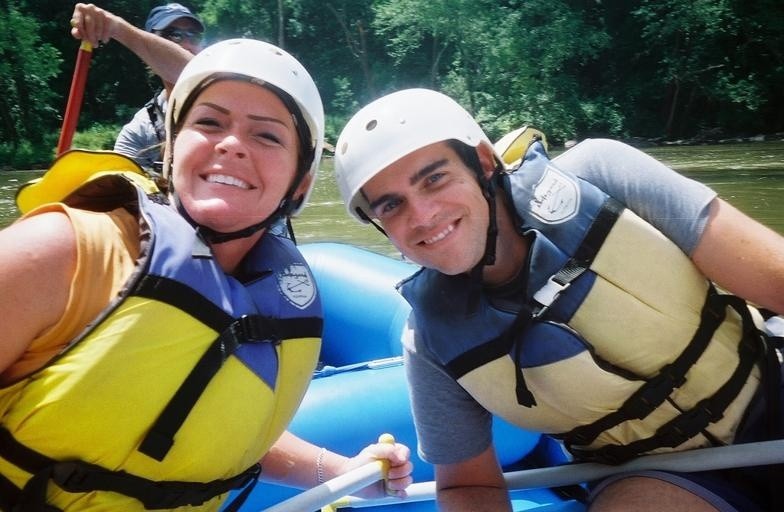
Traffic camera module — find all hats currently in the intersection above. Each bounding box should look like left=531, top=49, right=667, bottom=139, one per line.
left=146, top=4, right=204, bottom=34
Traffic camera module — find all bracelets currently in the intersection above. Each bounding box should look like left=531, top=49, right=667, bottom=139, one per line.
left=316, top=446, right=330, bottom=485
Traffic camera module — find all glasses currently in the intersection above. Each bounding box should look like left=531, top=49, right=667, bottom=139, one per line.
left=164, top=27, right=202, bottom=46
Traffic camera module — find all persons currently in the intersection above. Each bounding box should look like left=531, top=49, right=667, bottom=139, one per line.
left=0, top=38, right=414, bottom=512
left=332, top=87, right=784, bottom=512
left=70, top=3, right=204, bottom=185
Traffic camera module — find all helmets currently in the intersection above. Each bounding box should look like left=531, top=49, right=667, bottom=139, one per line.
left=162, top=38, right=325, bottom=216
left=334, top=88, right=506, bottom=225
